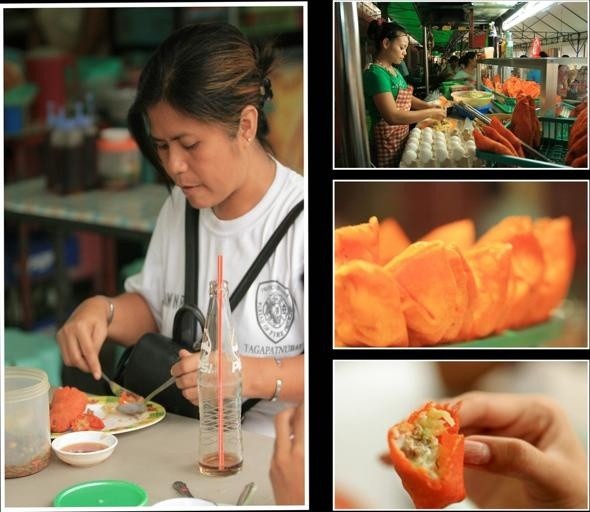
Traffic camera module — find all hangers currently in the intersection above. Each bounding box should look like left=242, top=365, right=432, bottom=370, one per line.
left=5, top=390, right=275, bottom=506
left=4, top=174, right=174, bottom=330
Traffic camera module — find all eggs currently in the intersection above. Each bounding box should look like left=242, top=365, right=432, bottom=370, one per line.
left=402, top=127, right=476, bottom=165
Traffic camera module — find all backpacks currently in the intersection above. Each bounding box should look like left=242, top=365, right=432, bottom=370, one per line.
left=106, top=197, right=304, bottom=426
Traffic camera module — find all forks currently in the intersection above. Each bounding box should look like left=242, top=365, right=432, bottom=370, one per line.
left=101, top=370, right=138, bottom=398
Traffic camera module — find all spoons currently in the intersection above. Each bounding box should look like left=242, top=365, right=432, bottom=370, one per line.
left=116, top=375, right=175, bottom=415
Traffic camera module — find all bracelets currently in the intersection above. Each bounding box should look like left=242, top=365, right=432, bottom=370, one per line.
left=104, top=295, right=118, bottom=324
left=270, top=355, right=283, bottom=401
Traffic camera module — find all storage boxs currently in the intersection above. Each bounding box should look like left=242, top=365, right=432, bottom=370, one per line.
left=442, top=81, right=464, bottom=99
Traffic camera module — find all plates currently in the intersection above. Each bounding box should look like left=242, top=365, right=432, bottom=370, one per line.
left=44, top=395, right=166, bottom=439
left=52, top=431, right=118, bottom=467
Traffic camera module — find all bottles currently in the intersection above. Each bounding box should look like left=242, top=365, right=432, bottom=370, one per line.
left=197, top=280, right=244, bottom=477
left=488, top=21, right=513, bottom=58
left=42, top=91, right=143, bottom=197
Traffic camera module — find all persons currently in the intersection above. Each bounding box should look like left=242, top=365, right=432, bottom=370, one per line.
left=269, top=407, right=305, bottom=506
left=521, top=50, right=578, bottom=97
left=58, top=24, right=305, bottom=407
left=382, top=383, right=585, bottom=506
left=363, top=17, right=446, bottom=166
left=438, top=49, right=479, bottom=82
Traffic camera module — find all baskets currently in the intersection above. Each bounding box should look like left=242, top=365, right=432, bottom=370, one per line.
left=441, top=81, right=577, bottom=168
